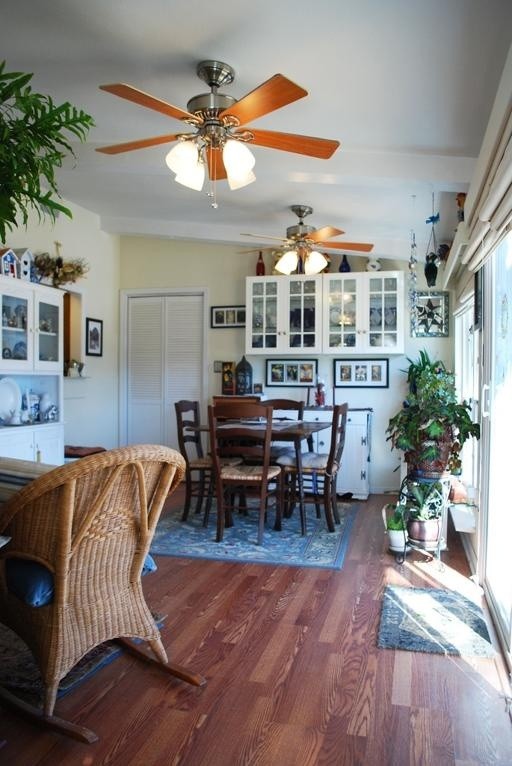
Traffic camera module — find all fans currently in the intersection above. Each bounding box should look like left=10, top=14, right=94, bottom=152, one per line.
left=237, top=205, right=374, bottom=255
left=94, top=61, right=341, bottom=160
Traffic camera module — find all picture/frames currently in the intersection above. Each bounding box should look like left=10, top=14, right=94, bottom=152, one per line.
left=85, top=317, right=103, bottom=356
left=210, top=306, right=246, bottom=328
left=333, top=358, right=389, bottom=389
left=417, top=290, right=449, bottom=337
left=266, top=359, right=318, bottom=387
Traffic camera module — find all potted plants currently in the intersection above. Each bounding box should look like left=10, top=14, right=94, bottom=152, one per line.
left=387, top=517, right=412, bottom=553
left=383, top=480, right=452, bottom=548
left=386, top=345, right=481, bottom=480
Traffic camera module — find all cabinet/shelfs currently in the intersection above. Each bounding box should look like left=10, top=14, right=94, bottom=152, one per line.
left=321, top=270, right=405, bottom=355
left=0, top=274, right=65, bottom=466
left=245, top=274, right=322, bottom=355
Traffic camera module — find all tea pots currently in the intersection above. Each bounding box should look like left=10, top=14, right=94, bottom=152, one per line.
left=372, top=335, right=395, bottom=346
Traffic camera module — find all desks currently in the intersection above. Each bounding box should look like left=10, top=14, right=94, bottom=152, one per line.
left=0, top=456, right=61, bottom=507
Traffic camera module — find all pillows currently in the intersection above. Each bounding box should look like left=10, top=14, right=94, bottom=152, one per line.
left=0, top=549, right=158, bottom=607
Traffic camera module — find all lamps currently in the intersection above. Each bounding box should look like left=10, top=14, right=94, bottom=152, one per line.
left=273, top=246, right=328, bottom=274
left=164, top=126, right=256, bottom=208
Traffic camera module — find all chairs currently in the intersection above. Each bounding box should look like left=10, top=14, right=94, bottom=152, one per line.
left=0, top=445, right=207, bottom=744
left=175, top=398, right=348, bottom=546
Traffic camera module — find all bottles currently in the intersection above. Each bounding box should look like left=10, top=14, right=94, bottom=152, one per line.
left=365, top=253, right=381, bottom=271
left=22, top=389, right=53, bottom=421
left=256, top=251, right=265, bottom=275
left=338, top=254, right=350, bottom=272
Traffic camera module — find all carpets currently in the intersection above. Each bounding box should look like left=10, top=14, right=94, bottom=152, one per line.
left=147, top=493, right=360, bottom=571
left=377, top=583, right=496, bottom=660
left=0, top=608, right=169, bottom=704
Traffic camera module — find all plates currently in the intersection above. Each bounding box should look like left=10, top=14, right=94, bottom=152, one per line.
left=0, top=377, right=23, bottom=421
left=14, top=341, right=27, bottom=359
left=3, top=348, right=11, bottom=358
left=329, top=308, right=355, bottom=327
